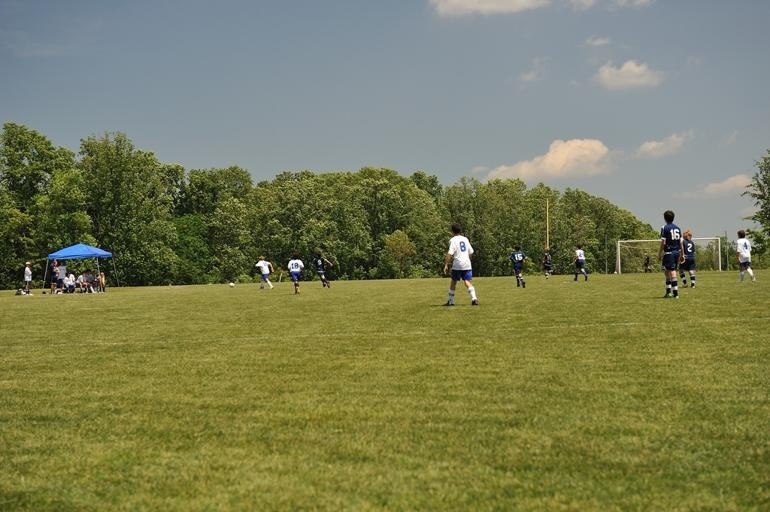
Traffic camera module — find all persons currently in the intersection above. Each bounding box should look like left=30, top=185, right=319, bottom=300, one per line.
left=736, top=230, right=756, bottom=282
left=657, top=211, right=686, bottom=299
left=288, top=253, right=305, bottom=294
left=444, top=223, right=480, bottom=306
left=574, top=245, right=588, bottom=281
left=544, top=247, right=552, bottom=279
left=678, top=230, right=696, bottom=289
left=50, top=258, right=106, bottom=296
left=255, top=255, right=275, bottom=290
left=644, top=253, right=652, bottom=273
left=313, top=252, right=333, bottom=288
left=510, top=245, right=528, bottom=288
left=25, top=262, right=33, bottom=295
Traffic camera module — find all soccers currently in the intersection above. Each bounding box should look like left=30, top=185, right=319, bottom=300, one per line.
left=229, top=283, right=235, bottom=288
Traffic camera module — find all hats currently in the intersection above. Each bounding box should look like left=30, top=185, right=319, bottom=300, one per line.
left=26, top=262, right=30, bottom=265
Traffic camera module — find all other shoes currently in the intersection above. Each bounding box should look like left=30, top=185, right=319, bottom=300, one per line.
left=442, top=301, right=454, bottom=306
left=523, top=282, right=525, bottom=288
left=672, top=294, right=679, bottom=299
left=471, top=300, right=478, bottom=305
left=295, top=287, right=301, bottom=294
left=585, top=275, right=588, bottom=281
left=323, top=281, right=330, bottom=287
left=663, top=293, right=672, bottom=298
left=680, top=284, right=688, bottom=288
left=691, top=285, right=696, bottom=288
